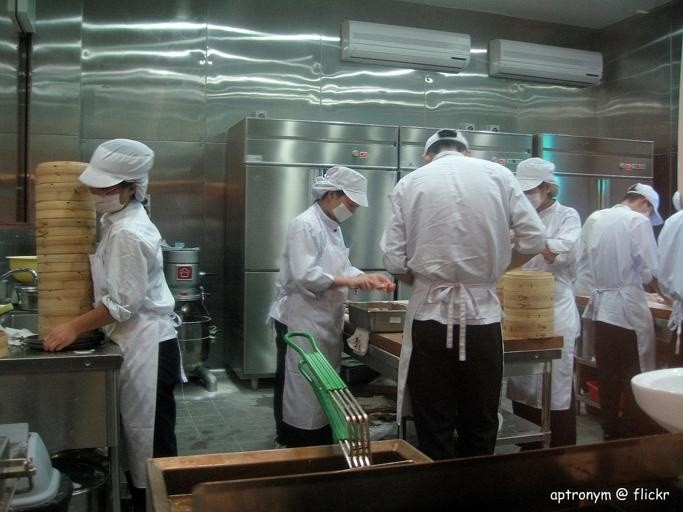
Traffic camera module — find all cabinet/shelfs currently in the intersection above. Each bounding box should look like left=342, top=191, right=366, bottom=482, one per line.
left=573, top=304, right=624, bottom=417
left=340, top=327, right=563, bottom=456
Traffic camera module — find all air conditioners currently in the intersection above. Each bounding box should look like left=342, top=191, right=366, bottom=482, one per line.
left=483, top=37, right=605, bottom=90
left=336, top=18, right=473, bottom=75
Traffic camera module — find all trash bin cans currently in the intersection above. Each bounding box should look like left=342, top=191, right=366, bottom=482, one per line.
left=0, top=433, right=72, bottom=511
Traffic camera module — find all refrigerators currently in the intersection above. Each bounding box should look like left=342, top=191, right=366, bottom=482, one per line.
left=535, top=132, right=656, bottom=224
left=219, top=117, right=399, bottom=391
left=398, top=124, right=534, bottom=302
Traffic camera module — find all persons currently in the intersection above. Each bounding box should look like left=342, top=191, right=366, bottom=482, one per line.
left=657, top=210, right=683, bottom=369
left=505, top=155, right=582, bottom=451
left=40, top=137, right=190, bottom=512
left=578, top=182, right=666, bottom=442
left=380, top=128, right=545, bottom=461
left=264, top=163, right=396, bottom=449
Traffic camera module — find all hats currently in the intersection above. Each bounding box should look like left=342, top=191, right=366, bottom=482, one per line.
left=425, top=129, right=468, bottom=155
left=627, top=183, right=663, bottom=226
left=312, top=167, right=368, bottom=206
left=78, top=139, right=153, bottom=201
left=516, top=158, right=555, bottom=191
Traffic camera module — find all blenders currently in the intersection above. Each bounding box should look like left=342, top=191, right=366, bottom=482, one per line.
left=162, top=240, right=219, bottom=393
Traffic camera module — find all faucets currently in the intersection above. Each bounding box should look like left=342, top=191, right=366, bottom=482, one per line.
left=1, top=266, right=39, bottom=287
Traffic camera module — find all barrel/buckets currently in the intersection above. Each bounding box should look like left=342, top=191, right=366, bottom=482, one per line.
left=162, top=249, right=200, bottom=301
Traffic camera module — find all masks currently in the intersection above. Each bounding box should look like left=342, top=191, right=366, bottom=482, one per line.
left=330, top=197, right=352, bottom=223
left=527, top=188, right=548, bottom=208
left=90, top=193, right=127, bottom=212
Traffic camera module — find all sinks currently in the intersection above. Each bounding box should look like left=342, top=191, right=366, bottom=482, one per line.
left=0, top=311, right=40, bottom=345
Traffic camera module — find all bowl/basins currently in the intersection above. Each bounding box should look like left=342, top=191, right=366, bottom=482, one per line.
left=6, top=254, right=40, bottom=311
